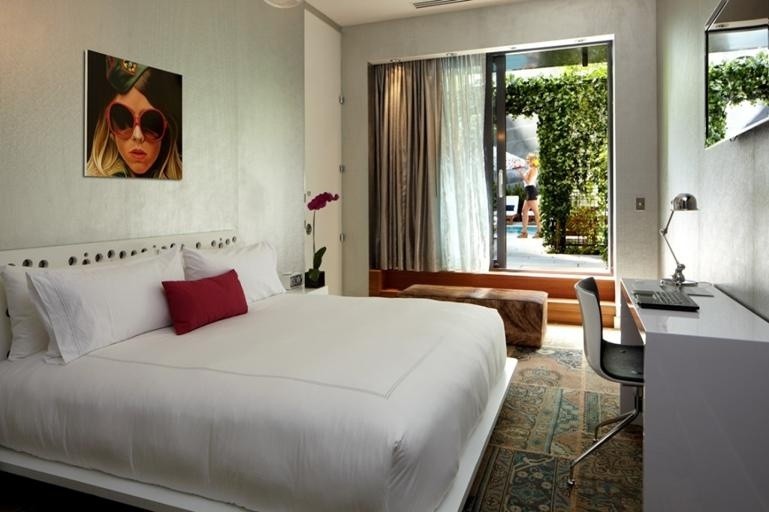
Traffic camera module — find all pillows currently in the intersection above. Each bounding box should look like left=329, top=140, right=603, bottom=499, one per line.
left=1, top=241, right=287, bottom=365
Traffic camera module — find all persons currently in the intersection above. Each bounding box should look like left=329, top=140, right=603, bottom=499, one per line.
left=516, top=152, right=542, bottom=238
left=86, top=50, right=182, bottom=179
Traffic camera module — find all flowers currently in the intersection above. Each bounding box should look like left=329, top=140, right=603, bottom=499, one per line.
left=307, top=191, right=340, bottom=282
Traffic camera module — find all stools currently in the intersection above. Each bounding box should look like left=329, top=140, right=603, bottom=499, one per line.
left=398, top=283, right=549, bottom=348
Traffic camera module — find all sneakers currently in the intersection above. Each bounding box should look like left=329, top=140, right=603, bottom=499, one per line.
left=533, top=233, right=543, bottom=238
left=518, top=232, right=528, bottom=239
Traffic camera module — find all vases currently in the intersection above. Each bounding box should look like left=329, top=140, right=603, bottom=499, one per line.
left=305, top=271, right=325, bottom=288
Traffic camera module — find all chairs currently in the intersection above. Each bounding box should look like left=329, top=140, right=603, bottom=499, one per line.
left=566, top=277, right=643, bottom=486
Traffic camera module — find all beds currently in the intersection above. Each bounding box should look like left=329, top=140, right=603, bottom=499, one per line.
left=0, top=229, right=519, bottom=511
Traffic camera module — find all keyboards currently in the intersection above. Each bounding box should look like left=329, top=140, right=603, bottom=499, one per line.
left=632, top=289, right=700, bottom=311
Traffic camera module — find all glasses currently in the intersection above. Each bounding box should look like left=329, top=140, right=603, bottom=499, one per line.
left=106, top=103, right=166, bottom=141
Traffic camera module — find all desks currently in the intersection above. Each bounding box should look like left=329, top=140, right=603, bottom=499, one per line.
left=621, top=278, right=769, bottom=512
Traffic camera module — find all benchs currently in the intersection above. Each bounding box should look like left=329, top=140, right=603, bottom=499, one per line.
left=369, top=269, right=616, bottom=328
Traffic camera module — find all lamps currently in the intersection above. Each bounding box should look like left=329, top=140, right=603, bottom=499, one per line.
left=659, top=193, right=700, bottom=289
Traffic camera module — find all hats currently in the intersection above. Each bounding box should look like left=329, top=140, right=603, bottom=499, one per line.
left=105, top=54, right=151, bottom=95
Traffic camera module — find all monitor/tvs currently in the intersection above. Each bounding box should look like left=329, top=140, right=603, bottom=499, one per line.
left=702, top=0, right=769, bottom=151
